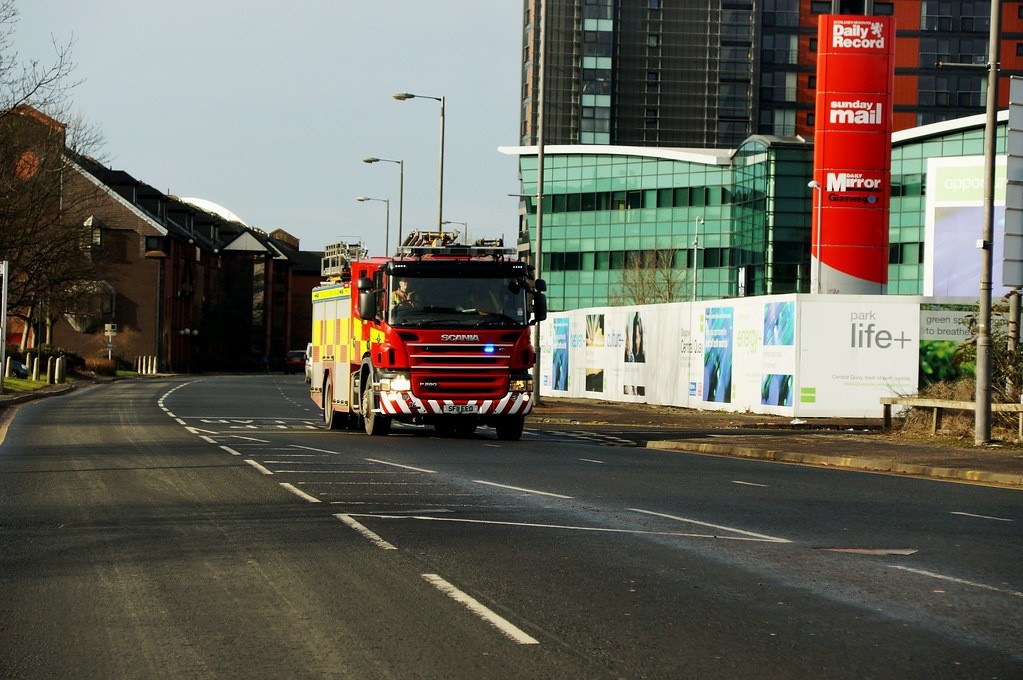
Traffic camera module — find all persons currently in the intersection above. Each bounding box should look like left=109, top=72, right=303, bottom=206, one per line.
left=625, top=314, right=645, bottom=364
left=392, top=278, right=419, bottom=311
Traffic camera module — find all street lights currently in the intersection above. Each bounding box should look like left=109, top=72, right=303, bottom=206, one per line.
left=441, top=220, right=468, bottom=244
left=808, top=180, right=823, bottom=293
left=363, top=157, right=403, bottom=247
left=391, top=91, right=445, bottom=235
left=693, top=215, right=705, bottom=300
left=356, top=196, right=389, bottom=257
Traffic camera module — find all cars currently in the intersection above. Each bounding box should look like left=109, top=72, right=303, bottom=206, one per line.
left=5, top=359, right=29, bottom=379
left=284, top=349, right=305, bottom=375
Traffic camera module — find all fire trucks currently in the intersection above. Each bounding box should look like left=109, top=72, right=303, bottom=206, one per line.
left=311, top=228, right=548, bottom=441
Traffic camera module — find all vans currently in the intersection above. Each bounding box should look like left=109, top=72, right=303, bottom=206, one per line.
left=304, top=342, right=312, bottom=384
